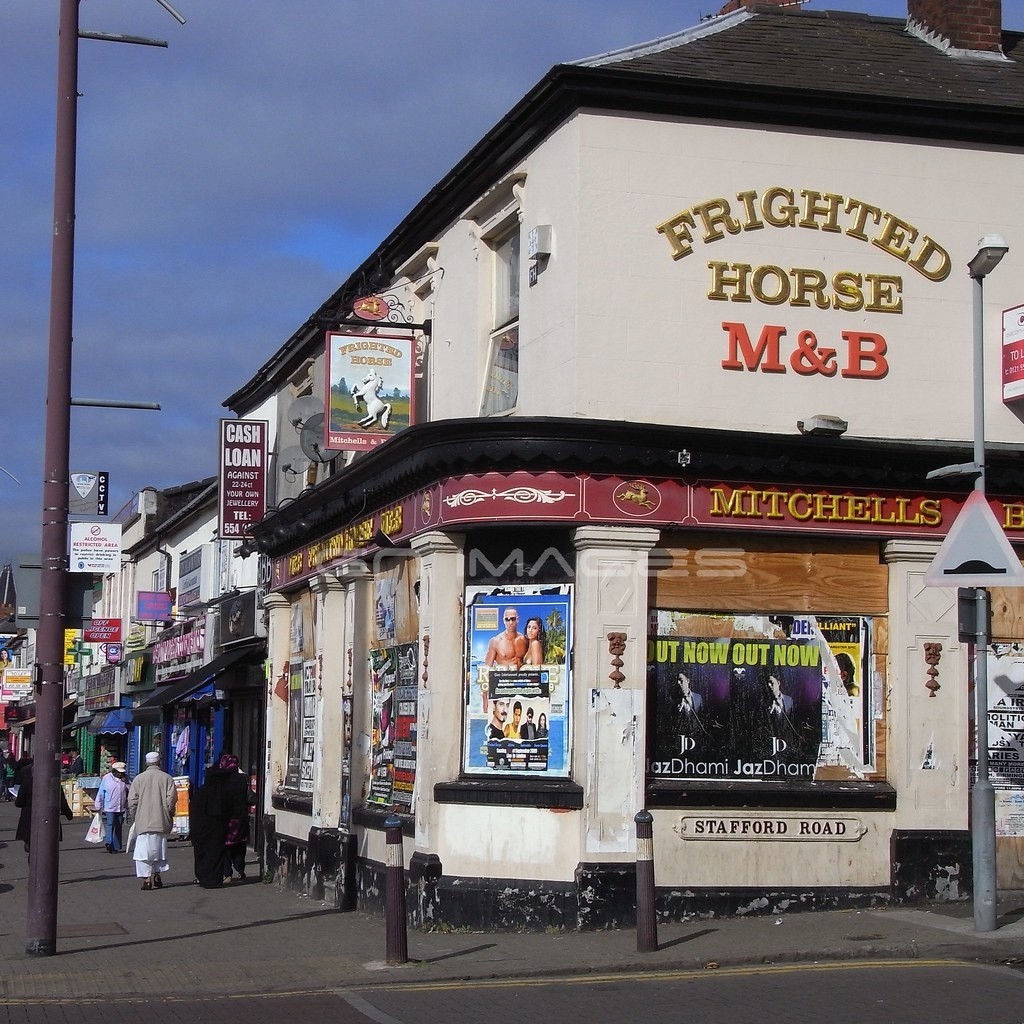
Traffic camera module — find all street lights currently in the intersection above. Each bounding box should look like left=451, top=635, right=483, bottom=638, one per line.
left=925, top=233, right=1010, bottom=934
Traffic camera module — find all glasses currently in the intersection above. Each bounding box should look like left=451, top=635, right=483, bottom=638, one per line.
left=505, top=617, right=516, bottom=621
left=529, top=716, right=532, bottom=718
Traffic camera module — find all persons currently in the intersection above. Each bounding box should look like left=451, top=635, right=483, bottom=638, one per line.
left=0, top=749, right=255, bottom=889
left=481, top=607, right=856, bottom=742
left=0, top=648, right=13, bottom=669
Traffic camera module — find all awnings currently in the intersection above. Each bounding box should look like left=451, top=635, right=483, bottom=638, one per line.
left=134, top=643, right=262, bottom=713
left=62, top=716, right=93, bottom=730
left=89, top=707, right=135, bottom=736
left=8, top=697, right=77, bottom=732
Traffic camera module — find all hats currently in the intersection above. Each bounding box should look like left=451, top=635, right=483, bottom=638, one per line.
left=146, top=752, right=160, bottom=763
left=112, top=762, right=128, bottom=772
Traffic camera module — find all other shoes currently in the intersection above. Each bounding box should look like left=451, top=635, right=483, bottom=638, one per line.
left=141, top=882, right=152, bottom=890
left=193, top=878, right=200, bottom=884
left=106, top=846, right=112, bottom=853
left=113, top=851, right=117, bottom=854
left=238, top=873, right=246, bottom=879
left=223, top=876, right=232, bottom=883
left=154, top=882, right=162, bottom=888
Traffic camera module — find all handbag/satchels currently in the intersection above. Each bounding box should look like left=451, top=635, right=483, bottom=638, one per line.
left=126, top=822, right=136, bottom=854
left=85, top=813, right=106, bottom=843
